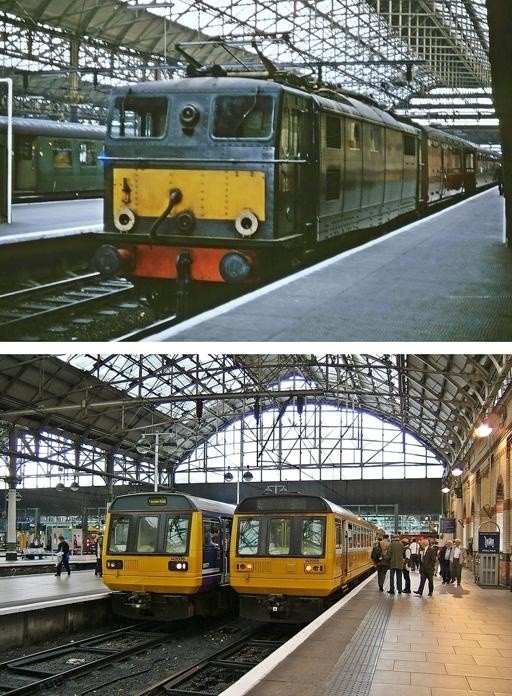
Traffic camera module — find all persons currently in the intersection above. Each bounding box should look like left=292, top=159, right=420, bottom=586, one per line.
left=494, top=161, right=504, bottom=195
left=55, top=535, right=70, bottom=576
left=94, top=539, right=102, bottom=577
left=371, top=534, right=465, bottom=596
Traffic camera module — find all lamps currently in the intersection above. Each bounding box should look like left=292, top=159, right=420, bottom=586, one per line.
left=136, top=432, right=178, bottom=454
left=474, top=405, right=507, bottom=437
left=225, top=464, right=254, bottom=482
left=452, top=457, right=471, bottom=476
left=440, top=476, right=457, bottom=496
left=55, top=474, right=80, bottom=493
left=6, top=490, right=22, bottom=502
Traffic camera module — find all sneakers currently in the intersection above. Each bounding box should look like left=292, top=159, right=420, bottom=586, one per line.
left=55, top=574, right=60, bottom=575
left=380, top=588, right=432, bottom=596
left=442, top=579, right=460, bottom=585
left=95, top=571, right=97, bottom=575
left=68, top=572, right=70, bottom=574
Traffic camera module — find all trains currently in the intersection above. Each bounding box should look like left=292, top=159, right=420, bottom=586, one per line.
left=95, top=77, right=500, bottom=313
left=1, top=116, right=138, bottom=200
left=101, top=492, right=385, bottom=623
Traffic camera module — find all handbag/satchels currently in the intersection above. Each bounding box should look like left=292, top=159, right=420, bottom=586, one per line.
left=56, top=552, right=64, bottom=567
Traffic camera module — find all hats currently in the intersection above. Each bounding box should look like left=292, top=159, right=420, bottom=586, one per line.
left=401, top=538, right=409, bottom=543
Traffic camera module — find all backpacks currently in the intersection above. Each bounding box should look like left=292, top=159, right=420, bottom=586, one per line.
left=371, top=546, right=382, bottom=561
